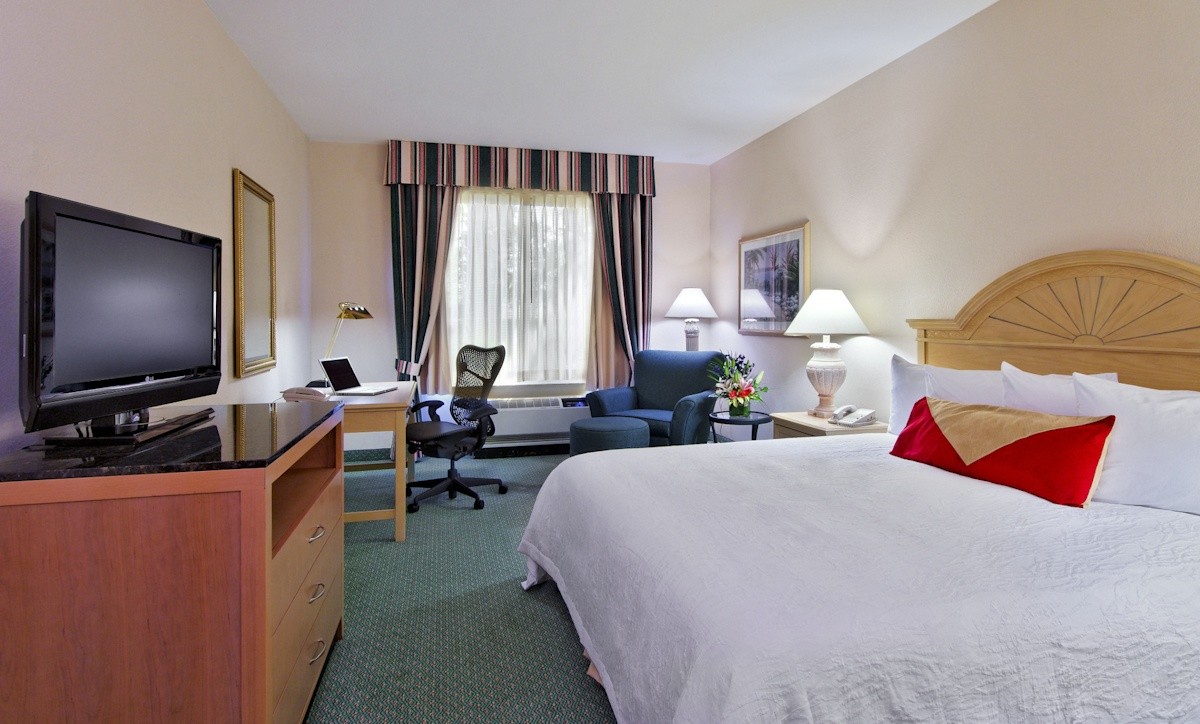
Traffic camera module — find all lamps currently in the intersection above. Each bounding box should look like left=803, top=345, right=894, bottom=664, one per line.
left=304, top=300, right=374, bottom=390
left=738, top=289, right=776, bottom=333
left=782, top=289, right=870, bottom=419
left=663, top=286, right=719, bottom=351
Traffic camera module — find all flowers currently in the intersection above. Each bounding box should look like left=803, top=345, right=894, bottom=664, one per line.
left=705, top=350, right=769, bottom=408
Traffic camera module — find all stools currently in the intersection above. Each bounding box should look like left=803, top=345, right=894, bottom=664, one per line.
left=565, top=415, right=650, bottom=457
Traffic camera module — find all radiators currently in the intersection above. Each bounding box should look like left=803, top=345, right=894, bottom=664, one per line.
left=480, top=395, right=591, bottom=450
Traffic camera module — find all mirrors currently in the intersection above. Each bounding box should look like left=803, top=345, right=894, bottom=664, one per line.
left=230, top=167, right=277, bottom=379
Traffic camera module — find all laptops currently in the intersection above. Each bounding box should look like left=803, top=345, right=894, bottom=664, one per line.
left=318, top=356, right=399, bottom=396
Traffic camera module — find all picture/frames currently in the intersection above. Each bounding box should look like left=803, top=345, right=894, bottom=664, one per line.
left=735, top=217, right=817, bottom=339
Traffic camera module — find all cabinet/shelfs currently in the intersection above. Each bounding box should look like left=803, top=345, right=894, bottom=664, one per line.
left=0, top=399, right=349, bottom=723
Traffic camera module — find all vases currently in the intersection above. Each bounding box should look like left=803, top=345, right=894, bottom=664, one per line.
left=726, top=401, right=751, bottom=419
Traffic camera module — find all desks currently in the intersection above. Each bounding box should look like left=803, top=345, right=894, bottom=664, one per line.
left=269, top=380, right=420, bottom=542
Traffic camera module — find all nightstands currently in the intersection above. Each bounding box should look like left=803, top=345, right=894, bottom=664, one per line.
left=765, top=411, right=889, bottom=439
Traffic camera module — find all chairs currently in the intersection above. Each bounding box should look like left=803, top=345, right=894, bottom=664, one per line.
left=405, top=343, right=511, bottom=514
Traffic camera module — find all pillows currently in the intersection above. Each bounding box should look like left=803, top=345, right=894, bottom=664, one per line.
left=888, top=396, right=1120, bottom=510
left=885, top=353, right=1119, bottom=437
left=1068, top=370, right=1200, bottom=519
left=997, top=359, right=1083, bottom=422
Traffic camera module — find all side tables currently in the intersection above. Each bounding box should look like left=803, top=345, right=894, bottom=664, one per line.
left=708, top=409, right=772, bottom=442
left=517, top=247, right=1200, bottom=724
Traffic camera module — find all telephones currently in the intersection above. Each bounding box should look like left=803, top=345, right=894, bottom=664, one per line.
left=832, top=405, right=879, bottom=427
left=283, top=387, right=330, bottom=402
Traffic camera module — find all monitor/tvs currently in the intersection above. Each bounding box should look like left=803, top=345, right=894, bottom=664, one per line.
left=19, top=190, right=222, bottom=435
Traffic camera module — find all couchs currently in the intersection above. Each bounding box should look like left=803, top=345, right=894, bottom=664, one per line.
left=585, top=347, right=730, bottom=446
